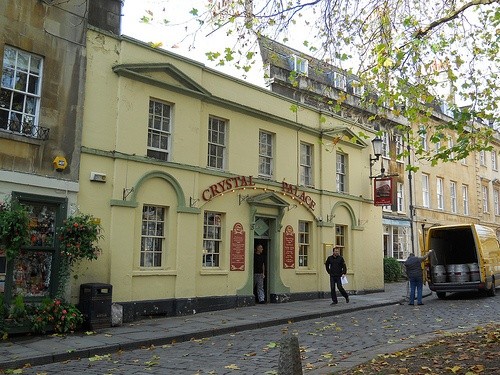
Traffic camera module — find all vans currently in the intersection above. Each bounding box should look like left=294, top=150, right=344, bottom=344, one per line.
left=418, top=223, right=500, bottom=299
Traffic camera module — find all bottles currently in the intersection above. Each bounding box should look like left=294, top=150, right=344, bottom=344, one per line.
left=276, top=296, right=278, bottom=303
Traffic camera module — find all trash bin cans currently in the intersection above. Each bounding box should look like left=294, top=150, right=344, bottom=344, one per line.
left=79, top=282, right=113, bottom=329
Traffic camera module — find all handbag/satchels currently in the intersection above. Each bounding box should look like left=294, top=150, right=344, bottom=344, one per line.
left=340, top=275, right=349, bottom=284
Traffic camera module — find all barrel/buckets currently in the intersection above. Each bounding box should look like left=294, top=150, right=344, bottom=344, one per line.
left=431, top=262, right=480, bottom=282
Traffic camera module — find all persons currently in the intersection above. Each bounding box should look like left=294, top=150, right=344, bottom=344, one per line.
left=404, top=249, right=433, bottom=306
left=254, top=244, right=265, bottom=304
left=326, top=247, right=349, bottom=306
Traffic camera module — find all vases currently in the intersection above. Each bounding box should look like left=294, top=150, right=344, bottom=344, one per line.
left=4, top=318, right=32, bottom=334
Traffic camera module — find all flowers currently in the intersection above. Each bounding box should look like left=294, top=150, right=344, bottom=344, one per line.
left=0, top=194, right=32, bottom=261
left=27, top=299, right=86, bottom=333
left=58, top=213, right=104, bottom=264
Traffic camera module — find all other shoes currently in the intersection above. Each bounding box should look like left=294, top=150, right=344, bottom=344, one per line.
left=346, top=296, right=350, bottom=303
left=408, top=303, right=414, bottom=305
left=417, top=303, right=424, bottom=306
left=258, top=301, right=266, bottom=304
left=331, top=299, right=338, bottom=305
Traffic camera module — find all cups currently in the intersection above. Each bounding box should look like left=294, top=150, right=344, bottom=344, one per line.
left=70, top=331, right=74, bottom=335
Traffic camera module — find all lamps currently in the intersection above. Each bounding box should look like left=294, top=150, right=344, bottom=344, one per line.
left=369, top=137, right=383, bottom=183
